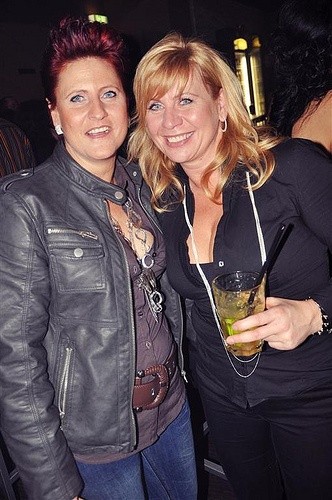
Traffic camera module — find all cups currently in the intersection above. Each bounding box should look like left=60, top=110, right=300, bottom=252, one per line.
left=212, top=272, right=265, bottom=356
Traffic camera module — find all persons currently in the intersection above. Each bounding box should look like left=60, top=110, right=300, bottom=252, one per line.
left=0, top=15, right=198, bottom=500
left=118, top=30, right=332, bottom=500
left=247, top=0, right=332, bottom=154
left=0, top=94, right=56, bottom=182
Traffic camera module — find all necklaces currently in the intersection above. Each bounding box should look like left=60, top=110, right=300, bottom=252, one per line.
left=104, top=198, right=165, bottom=322
left=180, top=171, right=271, bottom=379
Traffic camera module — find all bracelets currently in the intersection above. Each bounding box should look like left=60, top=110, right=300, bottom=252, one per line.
left=304, top=297, right=330, bottom=337
